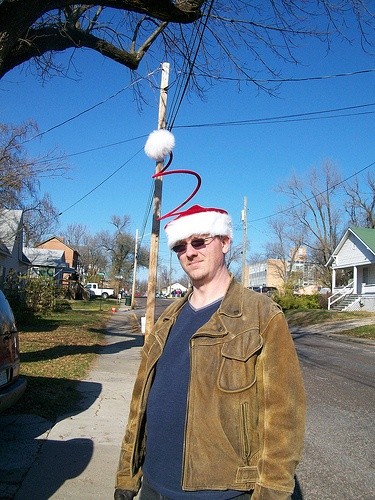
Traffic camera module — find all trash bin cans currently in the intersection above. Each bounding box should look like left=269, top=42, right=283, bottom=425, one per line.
left=125, top=296, right=131, bottom=306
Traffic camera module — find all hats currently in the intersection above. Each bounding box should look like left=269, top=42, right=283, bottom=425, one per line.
left=144, top=129, right=233, bottom=250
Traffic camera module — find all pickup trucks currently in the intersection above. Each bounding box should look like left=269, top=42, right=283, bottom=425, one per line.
left=83, top=283, right=115, bottom=298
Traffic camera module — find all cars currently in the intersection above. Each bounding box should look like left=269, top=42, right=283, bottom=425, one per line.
left=124, top=288, right=165, bottom=299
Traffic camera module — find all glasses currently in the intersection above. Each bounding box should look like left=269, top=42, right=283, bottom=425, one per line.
left=170, top=237, right=215, bottom=253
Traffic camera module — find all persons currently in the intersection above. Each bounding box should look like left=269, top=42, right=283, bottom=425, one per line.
left=112, top=129, right=305, bottom=500
